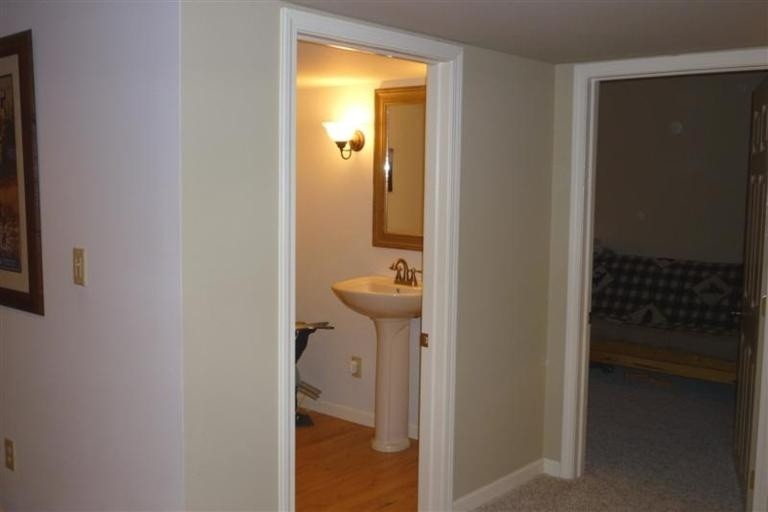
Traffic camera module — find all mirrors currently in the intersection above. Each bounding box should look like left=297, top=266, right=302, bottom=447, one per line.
left=372, top=86, right=425, bottom=252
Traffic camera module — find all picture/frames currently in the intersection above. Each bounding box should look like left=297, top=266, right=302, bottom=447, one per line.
left=0, top=28, right=44, bottom=317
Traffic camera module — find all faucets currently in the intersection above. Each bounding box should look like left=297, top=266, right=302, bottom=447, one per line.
left=393, top=258, right=409, bottom=283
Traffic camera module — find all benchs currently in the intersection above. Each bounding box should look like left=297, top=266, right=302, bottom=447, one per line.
left=590, top=249, right=740, bottom=383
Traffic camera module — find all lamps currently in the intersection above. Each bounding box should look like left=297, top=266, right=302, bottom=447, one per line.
left=321, top=121, right=365, bottom=160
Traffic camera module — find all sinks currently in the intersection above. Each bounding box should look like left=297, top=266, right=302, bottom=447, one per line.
left=331, top=277, right=422, bottom=316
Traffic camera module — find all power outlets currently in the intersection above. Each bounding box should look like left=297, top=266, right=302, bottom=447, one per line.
left=352, top=357, right=362, bottom=379
